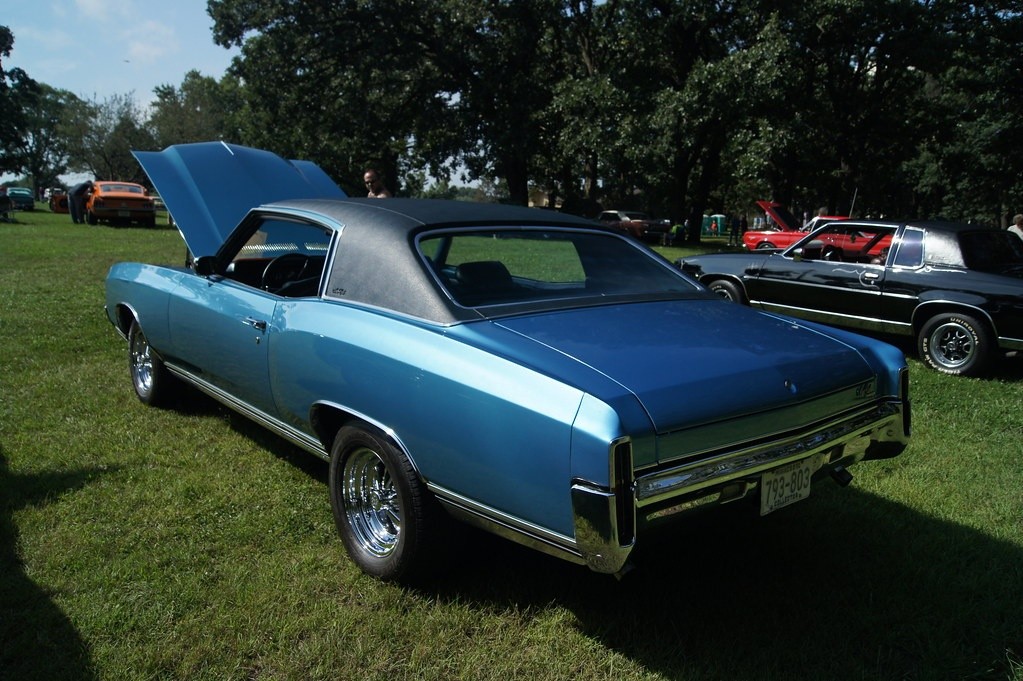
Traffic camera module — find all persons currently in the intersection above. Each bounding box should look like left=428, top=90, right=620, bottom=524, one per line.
left=66, top=179, right=95, bottom=225
left=1006, top=213, right=1022, bottom=242
left=362, top=168, right=393, bottom=198
left=617, top=207, right=759, bottom=247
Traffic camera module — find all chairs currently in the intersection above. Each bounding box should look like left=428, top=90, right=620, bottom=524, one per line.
left=455, top=260, right=529, bottom=308
left=869, top=246, right=889, bottom=265
left=270, top=276, right=321, bottom=299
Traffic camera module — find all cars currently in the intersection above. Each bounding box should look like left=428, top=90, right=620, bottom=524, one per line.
left=8, top=187, right=34, bottom=211
left=742, top=200, right=895, bottom=259
left=100, top=142, right=913, bottom=586
left=595, top=209, right=668, bottom=242
left=675, top=215, right=1023, bottom=377
left=87, top=180, right=157, bottom=229
